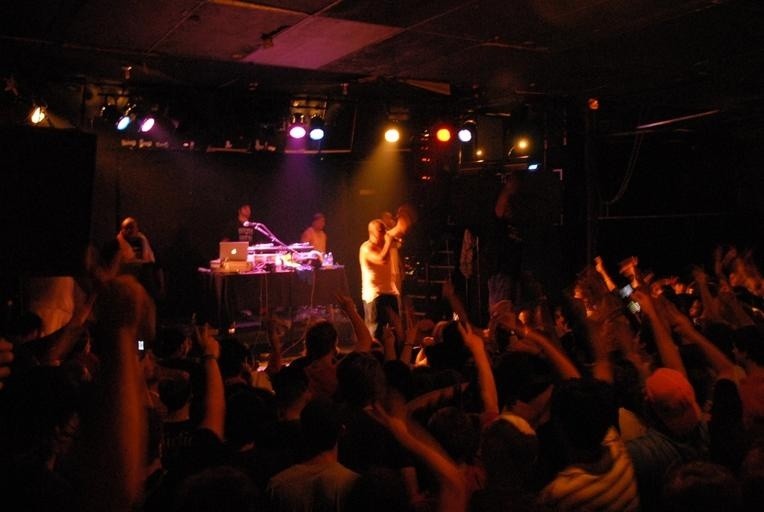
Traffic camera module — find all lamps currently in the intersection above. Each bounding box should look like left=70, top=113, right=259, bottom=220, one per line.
left=288, top=112, right=326, bottom=142
left=100, top=93, right=154, bottom=133
left=456, top=112, right=480, bottom=144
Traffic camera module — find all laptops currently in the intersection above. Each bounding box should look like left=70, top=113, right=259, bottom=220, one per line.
left=219, top=239, right=250, bottom=261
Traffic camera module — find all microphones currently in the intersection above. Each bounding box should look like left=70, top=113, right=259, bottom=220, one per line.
left=243, top=220, right=258, bottom=227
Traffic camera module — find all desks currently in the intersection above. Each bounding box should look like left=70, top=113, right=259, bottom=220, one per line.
left=198, top=264, right=346, bottom=330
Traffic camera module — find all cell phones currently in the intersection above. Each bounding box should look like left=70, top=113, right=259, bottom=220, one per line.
left=135, top=340, right=145, bottom=359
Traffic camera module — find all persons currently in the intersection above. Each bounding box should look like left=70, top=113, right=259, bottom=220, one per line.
left=379, top=211, right=410, bottom=316
left=97, top=217, right=166, bottom=306
left=297, top=212, right=328, bottom=255
left=357, top=215, right=408, bottom=337
left=223, top=203, right=256, bottom=246
